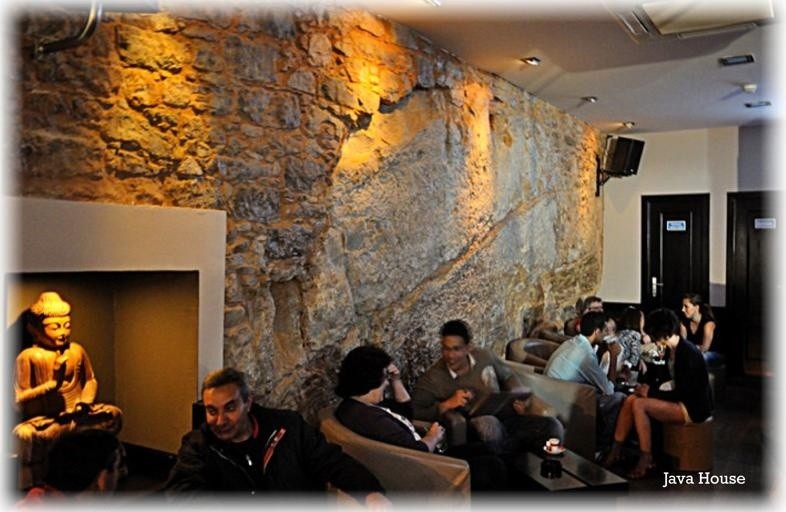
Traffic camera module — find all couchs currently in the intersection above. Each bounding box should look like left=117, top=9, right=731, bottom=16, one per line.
left=319, top=403, right=473, bottom=511
left=500, top=359, right=596, bottom=459
left=504, top=318, right=715, bottom=411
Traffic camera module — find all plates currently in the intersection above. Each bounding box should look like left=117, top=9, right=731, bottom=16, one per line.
left=541, top=447, right=565, bottom=456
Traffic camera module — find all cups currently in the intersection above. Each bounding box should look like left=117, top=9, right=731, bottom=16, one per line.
left=424, top=422, right=448, bottom=453
left=546, top=438, right=559, bottom=451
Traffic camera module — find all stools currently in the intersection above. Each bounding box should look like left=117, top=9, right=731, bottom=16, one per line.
left=663, top=417, right=721, bottom=474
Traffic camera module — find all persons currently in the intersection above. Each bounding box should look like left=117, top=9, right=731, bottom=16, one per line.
left=17, top=426, right=123, bottom=510
left=335, top=294, right=722, bottom=478
left=156, top=370, right=393, bottom=510
left=13, top=291, right=125, bottom=491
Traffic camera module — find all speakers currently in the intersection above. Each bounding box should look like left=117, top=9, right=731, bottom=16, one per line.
left=599, top=134, right=645, bottom=176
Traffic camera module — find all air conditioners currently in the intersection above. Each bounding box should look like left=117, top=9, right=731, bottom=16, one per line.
left=604, top=1, right=775, bottom=45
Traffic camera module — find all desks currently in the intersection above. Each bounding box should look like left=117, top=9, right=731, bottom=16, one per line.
left=518, top=438, right=627, bottom=493
